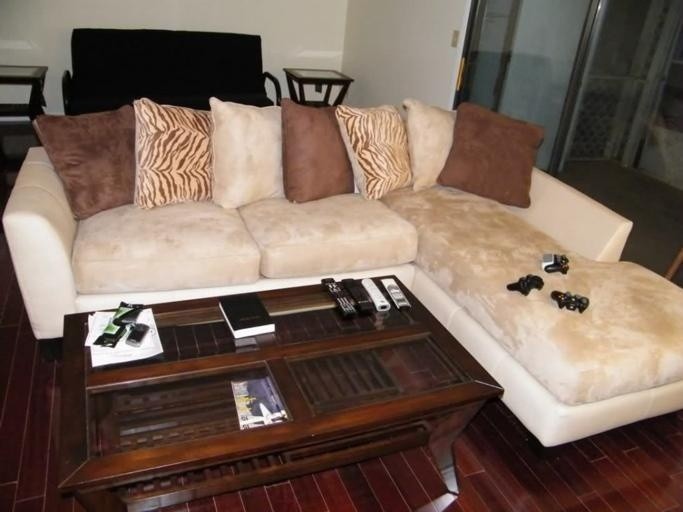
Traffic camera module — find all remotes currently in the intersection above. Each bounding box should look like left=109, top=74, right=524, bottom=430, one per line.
left=381, top=278, right=413, bottom=310
left=341, top=279, right=374, bottom=315
left=361, top=278, right=391, bottom=312
left=321, top=277, right=357, bottom=318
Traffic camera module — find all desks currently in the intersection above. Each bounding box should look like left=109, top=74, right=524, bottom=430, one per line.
left=0, top=65, right=49, bottom=171
left=283, top=67, right=354, bottom=107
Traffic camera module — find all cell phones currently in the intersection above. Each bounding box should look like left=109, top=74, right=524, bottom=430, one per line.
left=126, top=323, right=149, bottom=346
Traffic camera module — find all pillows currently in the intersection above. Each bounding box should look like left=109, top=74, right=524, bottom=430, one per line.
left=402, top=97, right=456, bottom=193
left=334, top=104, right=413, bottom=199
left=31, top=105, right=135, bottom=219
left=208, top=95, right=286, bottom=209
left=134, top=98, right=213, bottom=209
left=438, top=101, right=543, bottom=209
left=279, top=99, right=353, bottom=204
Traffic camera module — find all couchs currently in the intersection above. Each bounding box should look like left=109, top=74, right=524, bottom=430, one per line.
left=2, top=146, right=683, bottom=457
left=61, top=28, right=280, bottom=115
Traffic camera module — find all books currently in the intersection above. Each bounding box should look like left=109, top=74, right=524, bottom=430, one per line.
left=218, top=293, right=276, bottom=339
